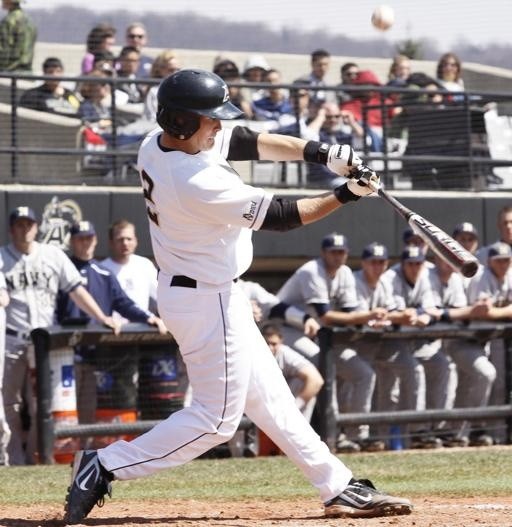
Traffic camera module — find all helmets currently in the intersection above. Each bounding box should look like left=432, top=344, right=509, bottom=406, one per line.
left=156, top=68, right=244, bottom=141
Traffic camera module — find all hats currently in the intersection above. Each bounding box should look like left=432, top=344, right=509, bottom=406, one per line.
left=11, top=206, right=37, bottom=228
left=322, top=223, right=512, bottom=262
left=70, top=221, right=95, bottom=236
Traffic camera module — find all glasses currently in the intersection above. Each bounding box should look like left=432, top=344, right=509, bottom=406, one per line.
left=129, top=34, right=360, bottom=118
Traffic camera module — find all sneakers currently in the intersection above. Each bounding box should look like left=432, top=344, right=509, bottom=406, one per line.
left=336, top=430, right=496, bottom=451
left=325, top=479, right=413, bottom=519
left=63, top=449, right=112, bottom=524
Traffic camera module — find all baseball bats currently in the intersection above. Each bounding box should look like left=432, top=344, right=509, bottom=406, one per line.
left=367, top=180, right=478, bottom=277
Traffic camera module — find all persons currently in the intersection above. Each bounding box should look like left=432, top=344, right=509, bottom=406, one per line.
left=19, top=57, right=81, bottom=116
left=65, top=67, right=415, bottom=525
left=228, top=207, right=511, bottom=466
left=4, top=2, right=34, bottom=71
left=4, top=198, right=168, bottom=465
left=75, top=22, right=466, bottom=172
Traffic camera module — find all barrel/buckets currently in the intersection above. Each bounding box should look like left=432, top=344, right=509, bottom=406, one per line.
left=19, top=346, right=189, bottom=465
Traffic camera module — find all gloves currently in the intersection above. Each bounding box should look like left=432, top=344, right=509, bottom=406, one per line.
left=347, top=165, right=380, bottom=197
left=317, top=142, right=362, bottom=179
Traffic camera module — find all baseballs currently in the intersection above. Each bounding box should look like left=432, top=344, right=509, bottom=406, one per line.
left=371, top=14, right=391, bottom=30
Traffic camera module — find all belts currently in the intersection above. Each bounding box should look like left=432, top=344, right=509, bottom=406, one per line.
left=6, top=327, right=31, bottom=341
left=157, top=268, right=238, bottom=288
